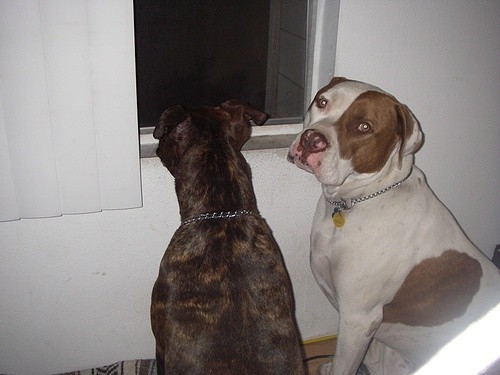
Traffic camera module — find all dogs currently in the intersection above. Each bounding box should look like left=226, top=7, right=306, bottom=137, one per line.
left=285, top=77, right=499, bottom=374
left=149, top=93, right=308, bottom=373
